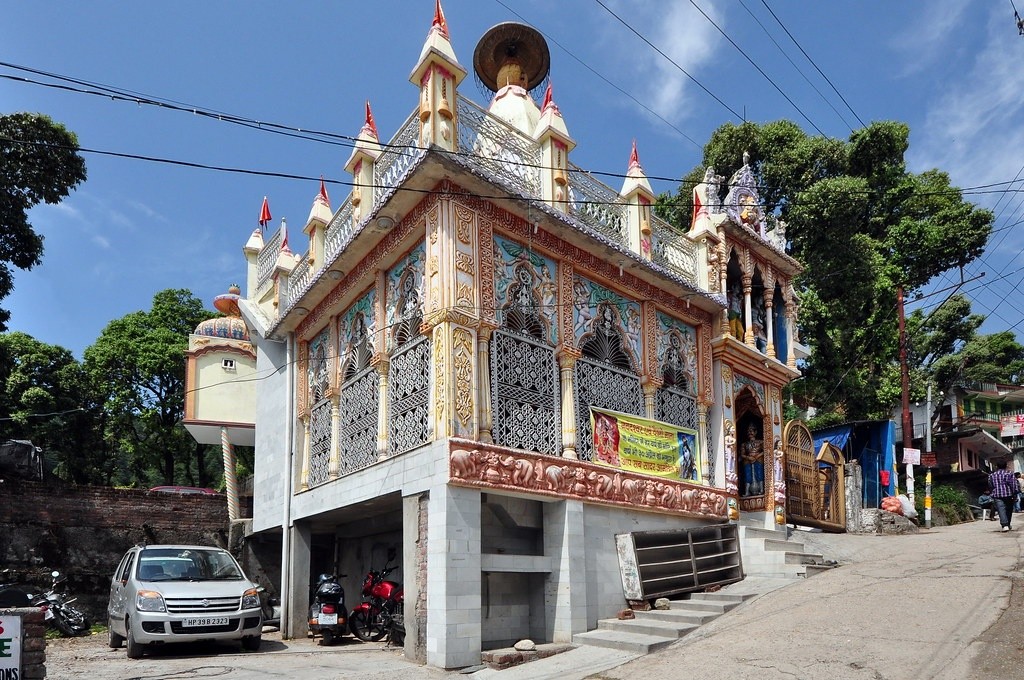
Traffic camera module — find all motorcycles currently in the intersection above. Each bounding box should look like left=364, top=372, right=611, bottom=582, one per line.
left=27, top=570, right=92, bottom=637
left=308, top=561, right=350, bottom=646
left=349, top=545, right=406, bottom=646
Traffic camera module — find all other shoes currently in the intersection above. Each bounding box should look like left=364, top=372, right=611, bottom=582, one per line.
left=1002, top=526, right=1009, bottom=531
left=991, top=518, right=996, bottom=521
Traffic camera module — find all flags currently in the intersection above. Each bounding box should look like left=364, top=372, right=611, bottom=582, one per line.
left=432, top=0, right=447, bottom=32
left=320, top=177, right=328, bottom=199
left=628, top=138, right=638, bottom=166
left=541, top=70, right=552, bottom=113
left=693, top=189, right=702, bottom=218
left=259, top=196, right=272, bottom=231
left=365, top=104, right=376, bottom=133
left=281, top=232, right=292, bottom=252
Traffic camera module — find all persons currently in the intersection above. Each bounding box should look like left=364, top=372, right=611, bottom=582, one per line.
left=338, top=316, right=348, bottom=347
left=680, top=332, right=697, bottom=365
left=740, top=423, right=764, bottom=497
left=533, top=265, right=555, bottom=320
left=656, top=326, right=672, bottom=361
left=725, top=425, right=736, bottom=475
left=625, top=306, right=641, bottom=362
left=776, top=216, right=790, bottom=254
left=773, top=440, right=784, bottom=482
left=368, top=297, right=378, bottom=340
left=987, top=461, right=1020, bottom=532
left=573, top=279, right=593, bottom=339
left=493, top=248, right=522, bottom=300
left=254, top=567, right=278, bottom=621
left=388, top=279, right=398, bottom=326
left=729, top=310, right=744, bottom=342
left=702, top=165, right=725, bottom=213
left=979, top=470, right=1024, bottom=522
left=306, top=344, right=316, bottom=374
left=409, top=250, right=426, bottom=309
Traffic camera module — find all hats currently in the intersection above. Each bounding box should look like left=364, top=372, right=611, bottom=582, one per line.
left=985, top=491, right=990, bottom=495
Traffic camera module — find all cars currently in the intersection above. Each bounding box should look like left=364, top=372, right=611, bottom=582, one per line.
left=146, top=486, right=219, bottom=497
left=108, top=541, right=266, bottom=660
